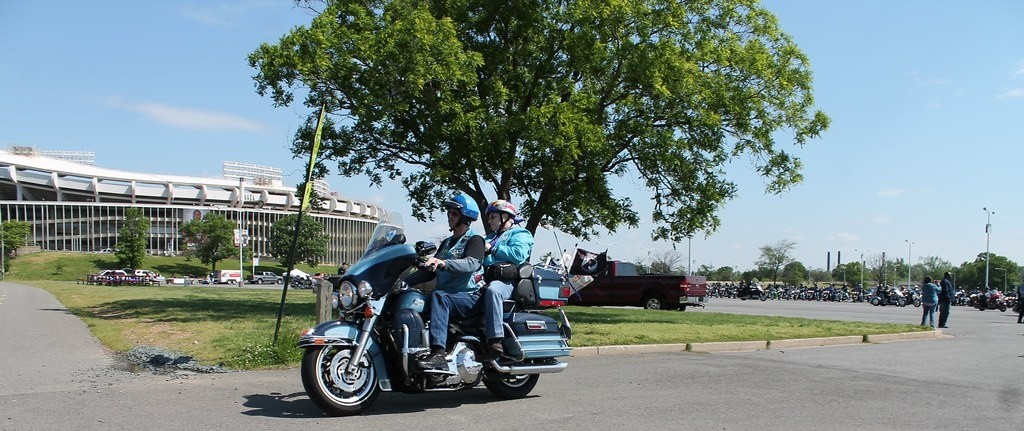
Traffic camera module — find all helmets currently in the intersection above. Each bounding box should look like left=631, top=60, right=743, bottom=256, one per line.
left=485, top=200, right=520, bottom=218
left=443, top=194, right=480, bottom=221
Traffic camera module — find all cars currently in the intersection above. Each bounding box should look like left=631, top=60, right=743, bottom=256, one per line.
left=92, top=249, right=119, bottom=254
left=97, top=270, right=129, bottom=281
left=133, top=270, right=162, bottom=278
left=118, top=268, right=135, bottom=274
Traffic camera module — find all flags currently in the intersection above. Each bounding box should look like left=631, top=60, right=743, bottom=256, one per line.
left=548, top=258, right=556, bottom=267
left=570, top=247, right=609, bottom=275
left=563, top=253, right=577, bottom=270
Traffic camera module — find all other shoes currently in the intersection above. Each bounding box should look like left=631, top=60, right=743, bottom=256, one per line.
left=489, top=341, right=504, bottom=353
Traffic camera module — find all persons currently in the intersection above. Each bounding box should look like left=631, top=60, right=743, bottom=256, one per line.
left=709, top=281, right=730, bottom=299
left=920, top=276, right=944, bottom=328
left=937, top=271, right=957, bottom=328
left=899, top=285, right=906, bottom=294
left=170, top=272, right=178, bottom=278
left=154, top=270, right=160, bottom=279
left=483, top=198, right=535, bottom=354
left=883, top=284, right=890, bottom=303
left=841, top=283, right=850, bottom=293
left=812, top=280, right=821, bottom=302
left=826, top=282, right=835, bottom=293
left=859, top=288, right=864, bottom=302
left=416, top=193, right=486, bottom=370
left=876, top=284, right=885, bottom=301
left=113, top=270, right=118, bottom=278
left=1016, top=286, right=1024, bottom=324
left=191, top=210, right=203, bottom=221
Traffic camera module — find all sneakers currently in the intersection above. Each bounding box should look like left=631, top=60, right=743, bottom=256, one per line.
left=417, top=346, right=449, bottom=370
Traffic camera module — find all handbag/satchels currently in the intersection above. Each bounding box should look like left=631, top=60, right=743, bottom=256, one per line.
left=488, top=262, right=517, bottom=281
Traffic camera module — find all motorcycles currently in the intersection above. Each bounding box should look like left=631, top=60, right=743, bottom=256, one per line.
left=871, top=289, right=1019, bottom=312
left=706, top=281, right=866, bottom=302
left=295, top=212, right=572, bottom=416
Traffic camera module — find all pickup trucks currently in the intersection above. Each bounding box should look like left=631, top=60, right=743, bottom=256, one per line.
left=247, top=271, right=283, bottom=285
left=569, top=261, right=708, bottom=312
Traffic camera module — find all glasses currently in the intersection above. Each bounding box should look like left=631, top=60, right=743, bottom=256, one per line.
left=490, top=214, right=498, bottom=220
left=448, top=210, right=460, bottom=217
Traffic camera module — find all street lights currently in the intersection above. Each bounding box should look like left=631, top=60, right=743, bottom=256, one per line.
left=687, top=234, right=694, bottom=276
left=597, top=242, right=618, bottom=261
left=906, top=240, right=914, bottom=290
left=951, top=273, right=955, bottom=290
left=891, top=264, right=900, bottom=287
left=855, top=249, right=869, bottom=288
left=983, top=208, right=995, bottom=288
left=995, top=268, right=1007, bottom=293
